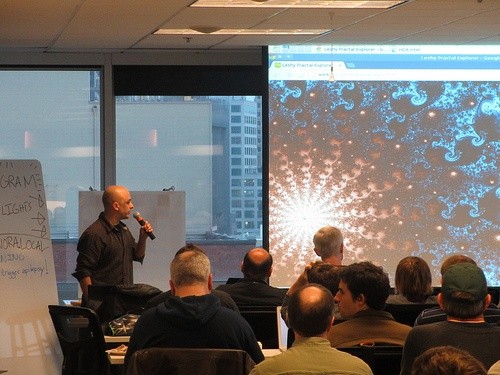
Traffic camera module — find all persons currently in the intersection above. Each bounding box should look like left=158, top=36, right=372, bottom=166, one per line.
left=405, top=262, right=500, bottom=375
left=214, top=248, right=287, bottom=349
left=328, top=262, right=413, bottom=349
left=281, top=226, right=352, bottom=329
left=414, top=255, right=500, bottom=326
left=248, top=283, right=374, bottom=375
left=144, top=243, right=240, bottom=314
left=411, top=346, right=488, bottom=375
left=385, top=257, right=438, bottom=304
left=125, top=251, right=265, bottom=375
left=71, top=186, right=164, bottom=322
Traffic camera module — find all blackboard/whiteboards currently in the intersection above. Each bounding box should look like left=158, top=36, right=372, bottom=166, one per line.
left=0, top=160, right=63, bottom=375
left=79, top=191, right=185, bottom=298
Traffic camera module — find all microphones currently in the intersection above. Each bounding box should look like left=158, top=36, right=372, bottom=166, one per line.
left=133, top=211, right=156, bottom=240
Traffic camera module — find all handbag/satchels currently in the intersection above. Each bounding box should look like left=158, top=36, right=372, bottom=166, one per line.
left=82, top=282, right=162, bottom=326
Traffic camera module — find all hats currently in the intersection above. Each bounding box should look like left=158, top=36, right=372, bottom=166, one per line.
left=442, top=262, right=487, bottom=305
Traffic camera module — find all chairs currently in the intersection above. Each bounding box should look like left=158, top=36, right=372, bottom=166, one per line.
left=47, top=277, right=440, bottom=375
